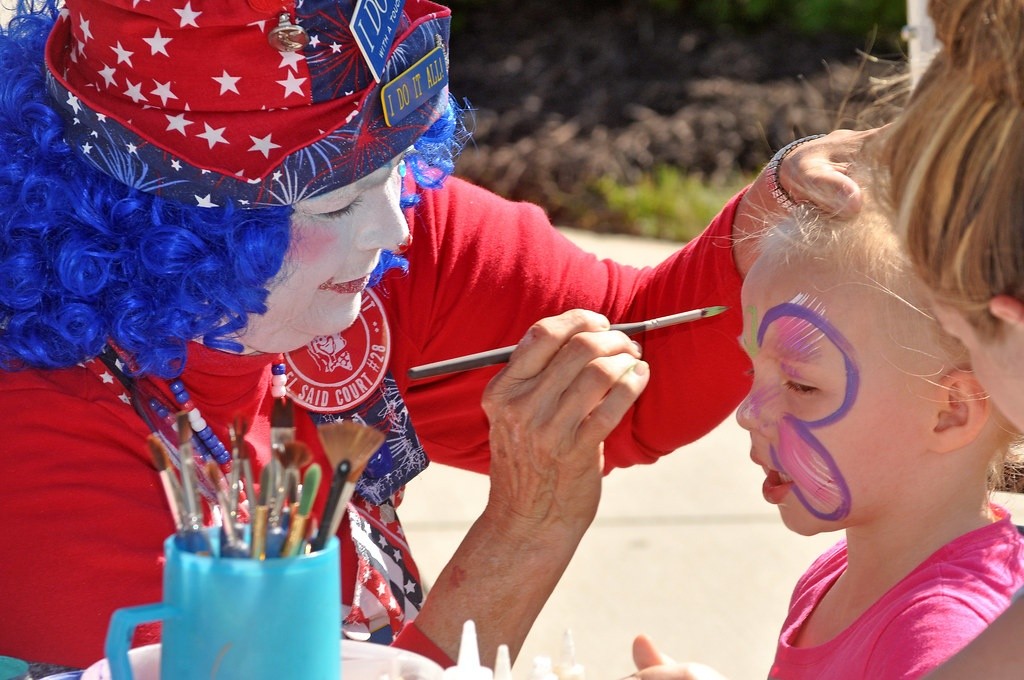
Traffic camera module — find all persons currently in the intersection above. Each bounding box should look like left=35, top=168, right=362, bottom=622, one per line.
left=863, top=0, right=1024, bottom=680
left=0, top=0, right=901, bottom=680
left=736, top=168, right=1024, bottom=680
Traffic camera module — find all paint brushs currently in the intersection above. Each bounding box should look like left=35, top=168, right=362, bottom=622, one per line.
left=143, top=410, right=389, bottom=564
left=406, top=306, right=730, bottom=378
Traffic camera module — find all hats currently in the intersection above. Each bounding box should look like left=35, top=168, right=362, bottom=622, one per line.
left=40, top=0, right=452, bottom=210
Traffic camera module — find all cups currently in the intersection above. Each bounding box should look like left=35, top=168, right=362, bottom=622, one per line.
left=105, top=522, right=342, bottom=679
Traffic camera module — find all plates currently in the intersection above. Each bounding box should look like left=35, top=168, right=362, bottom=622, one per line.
left=81, top=638, right=445, bottom=680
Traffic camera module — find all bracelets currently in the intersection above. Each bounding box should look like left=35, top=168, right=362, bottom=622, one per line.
left=767, top=132, right=828, bottom=216
